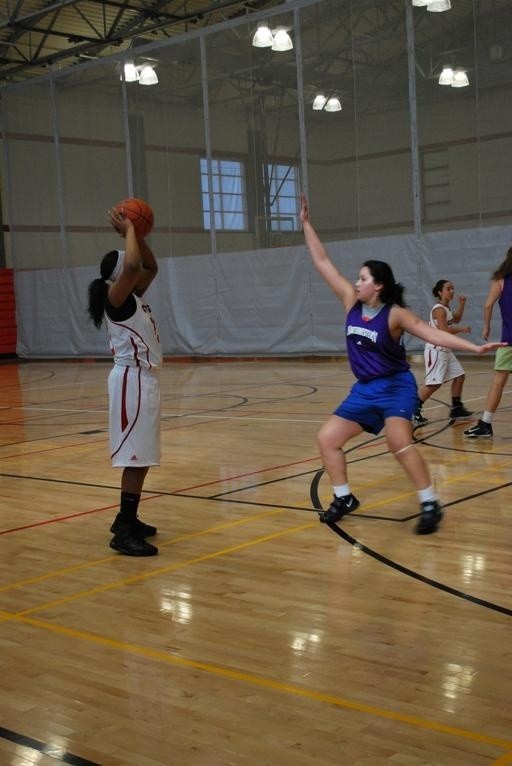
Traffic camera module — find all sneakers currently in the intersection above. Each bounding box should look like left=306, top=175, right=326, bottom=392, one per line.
left=110, top=532, right=158, bottom=556
left=111, top=512, right=156, bottom=538
left=463, top=419, right=492, bottom=437
left=414, top=407, right=427, bottom=424
left=320, top=493, right=359, bottom=523
left=449, top=407, right=474, bottom=418
left=415, top=501, right=443, bottom=535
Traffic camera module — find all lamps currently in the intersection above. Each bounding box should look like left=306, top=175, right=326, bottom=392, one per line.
left=411, top=0, right=452, bottom=12
left=309, top=84, right=350, bottom=113
left=248, top=15, right=296, bottom=51
left=110, top=53, right=167, bottom=86
left=428, top=54, right=473, bottom=88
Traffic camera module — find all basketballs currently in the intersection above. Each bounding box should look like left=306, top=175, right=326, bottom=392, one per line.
left=115, top=197, right=152, bottom=240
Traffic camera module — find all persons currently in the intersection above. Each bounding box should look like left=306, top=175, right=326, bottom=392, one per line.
left=413, top=279, right=476, bottom=425
left=298, top=190, right=510, bottom=535
left=88, top=203, right=163, bottom=557
left=463, top=245, right=512, bottom=438
